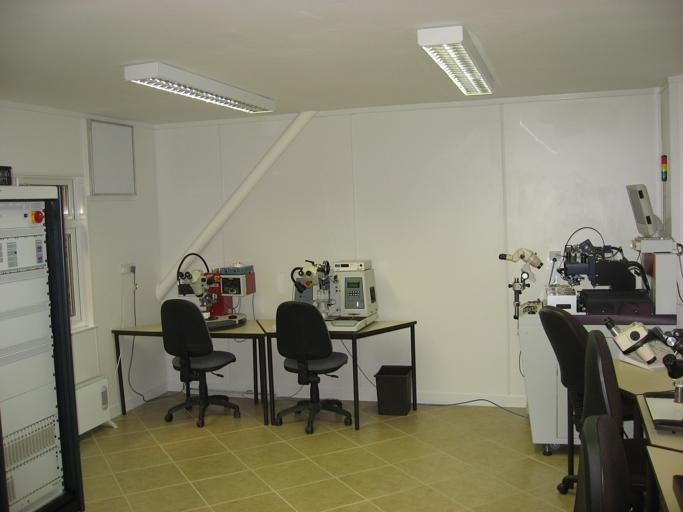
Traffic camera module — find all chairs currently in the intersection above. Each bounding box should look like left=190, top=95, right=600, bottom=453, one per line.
left=539, top=306, right=660, bottom=512
left=161, top=299, right=353, bottom=434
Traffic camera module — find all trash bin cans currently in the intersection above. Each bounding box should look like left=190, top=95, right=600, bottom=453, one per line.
left=373, top=364, right=413, bottom=416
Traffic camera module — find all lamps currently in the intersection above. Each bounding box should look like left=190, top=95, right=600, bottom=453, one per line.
left=128, top=63, right=277, bottom=114
left=416, top=27, right=495, bottom=97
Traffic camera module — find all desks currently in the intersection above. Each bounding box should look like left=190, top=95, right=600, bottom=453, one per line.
left=111, top=319, right=418, bottom=430
left=610, top=353, right=683, bottom=512
